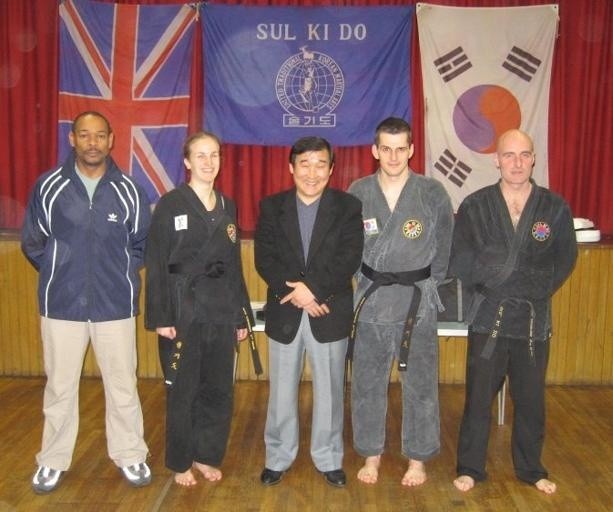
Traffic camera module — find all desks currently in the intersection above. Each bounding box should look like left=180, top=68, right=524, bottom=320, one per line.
left=2, top=221, right=612, bottom=385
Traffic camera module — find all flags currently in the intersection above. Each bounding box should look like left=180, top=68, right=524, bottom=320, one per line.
left=54, top=0, right=197, bottom=206
left=413, top=1, right=561, bottom=218
left=198, top=4, right=420, bottom=148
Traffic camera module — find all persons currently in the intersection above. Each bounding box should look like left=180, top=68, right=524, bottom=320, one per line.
left=443, top=126, right=579, bottom=495
left=250, top=134, right=366, bottom=489
left=344, top=113, right=456, bottom=490
left=141, top=129, right=257, bottom=489
left=18, top=110, right=154, bottom=495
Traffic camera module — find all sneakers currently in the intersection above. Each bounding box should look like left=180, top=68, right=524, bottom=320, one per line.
left=32, top=462, right=65, bottom=495
left=121, top=461, right=153, bottom=488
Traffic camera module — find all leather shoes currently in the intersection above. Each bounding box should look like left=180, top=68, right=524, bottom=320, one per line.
left=260, top=463, right=290, bottom=486
left=316, top=465, right=346, bottom=487
